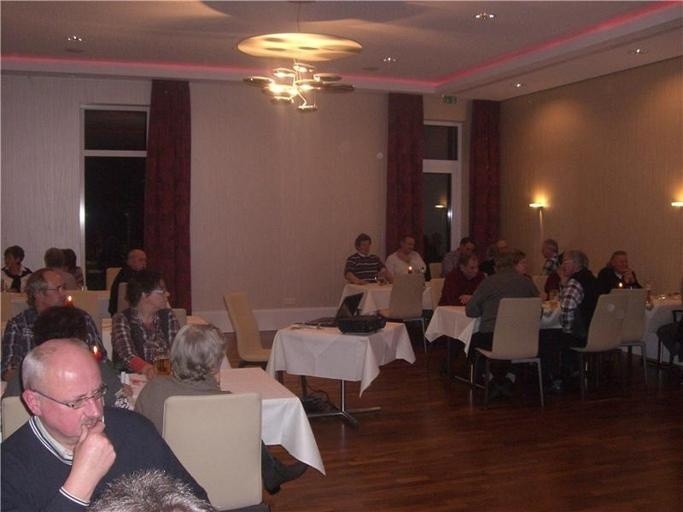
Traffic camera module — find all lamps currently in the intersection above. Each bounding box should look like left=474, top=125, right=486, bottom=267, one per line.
left=243, top=58, right=354, bottom=111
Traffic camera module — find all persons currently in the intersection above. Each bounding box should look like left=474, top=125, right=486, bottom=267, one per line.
left=4, top=268, right=133, bottom=410
left=466, top=252, right=541, bottom=386
left=0, top=338, right=215, bottom=512
left=1, top=246, right=85, bottom=293
left=135, top=323, right=308, bottom=512
left=541, top=238, right=643, bottom=388
left=108, top=249, right=180, bottom=378
left=439, top=238, right=512, bottom=376
left=345, top=233, right=427, bottom=315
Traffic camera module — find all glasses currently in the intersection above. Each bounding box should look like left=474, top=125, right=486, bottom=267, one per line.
left=152, top=287, right=168, bottom=295
left=45, top=284, right=65, bottom=294
left=29, top=384, right=109, bottom=409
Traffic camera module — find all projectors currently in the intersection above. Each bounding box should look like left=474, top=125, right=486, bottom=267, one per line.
left=339, top=314, right=386, bottom=334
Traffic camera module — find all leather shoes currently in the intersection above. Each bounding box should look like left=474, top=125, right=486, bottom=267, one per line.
left=265, top=457, right=309, bottom=495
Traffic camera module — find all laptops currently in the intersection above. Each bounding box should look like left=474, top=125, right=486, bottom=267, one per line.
left=305, top=292, right=364, bottom=327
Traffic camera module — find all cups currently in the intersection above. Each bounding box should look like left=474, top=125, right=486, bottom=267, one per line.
left=549, top=289, right=559, bottom=308
left=152, top=347, right=170, bottom=373
left=378, top=272, right=384, bottom=286
